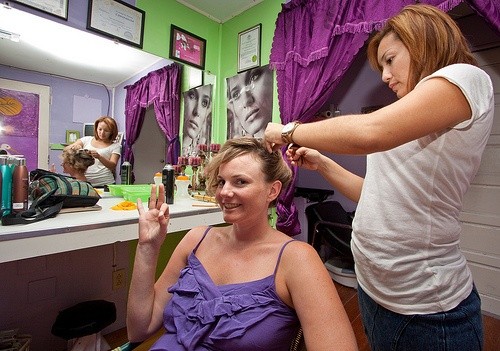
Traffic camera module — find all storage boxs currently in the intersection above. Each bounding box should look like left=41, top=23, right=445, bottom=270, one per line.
left=122, top=187, right=157, bottom=201
left=109, top=184, right=125, bottom=197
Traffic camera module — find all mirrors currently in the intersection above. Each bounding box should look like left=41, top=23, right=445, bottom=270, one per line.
left=0, top=4, right=217, bottom=186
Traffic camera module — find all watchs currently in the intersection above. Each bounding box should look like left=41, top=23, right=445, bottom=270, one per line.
left=281, top=120, right=302, bottom=144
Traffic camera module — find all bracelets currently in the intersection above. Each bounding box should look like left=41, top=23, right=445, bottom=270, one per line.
left=290, top=123, right=300, bottom=144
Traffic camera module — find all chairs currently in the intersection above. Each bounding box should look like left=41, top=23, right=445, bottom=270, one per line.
left=305, top=201, right=358, bottom=288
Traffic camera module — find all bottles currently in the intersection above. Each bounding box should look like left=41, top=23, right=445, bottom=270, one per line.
left=121, top=159, right=132, bottom=185
left=162, top=162, right=176, bottom=204
left=175, top=171, right=189, bottom=204
left=154, top=171, right=163, bottom=199
left=0, top=156, right=29, bottom=215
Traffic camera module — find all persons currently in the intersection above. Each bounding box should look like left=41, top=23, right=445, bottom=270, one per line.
left=264, top=6, right=494, bottom=351
left=179, top=84, right=212, bottom=153
left=126, top=137, right=359, bottom=351
left=228, top=65, right=272, bottom=137
left=68, top=114, right=121, bottom=188
left=48, top=145, right=96, bottom=189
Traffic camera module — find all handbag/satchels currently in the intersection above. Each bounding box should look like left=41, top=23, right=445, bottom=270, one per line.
left=16, top=169, right=100, bottom=225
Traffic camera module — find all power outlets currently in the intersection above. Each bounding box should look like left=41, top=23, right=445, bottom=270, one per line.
left=113, top=268, right=128, bottom=290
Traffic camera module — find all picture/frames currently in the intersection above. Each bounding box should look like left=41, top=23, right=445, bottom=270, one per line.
left=67, top=130, right=80, bottom=144
left=169, top=24, right=207, bottom=70
left=0, top=78, right=52, bottom=176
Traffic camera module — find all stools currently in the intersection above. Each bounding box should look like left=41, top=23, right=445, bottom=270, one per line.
left=51, top=299, right=117, bottom=351
